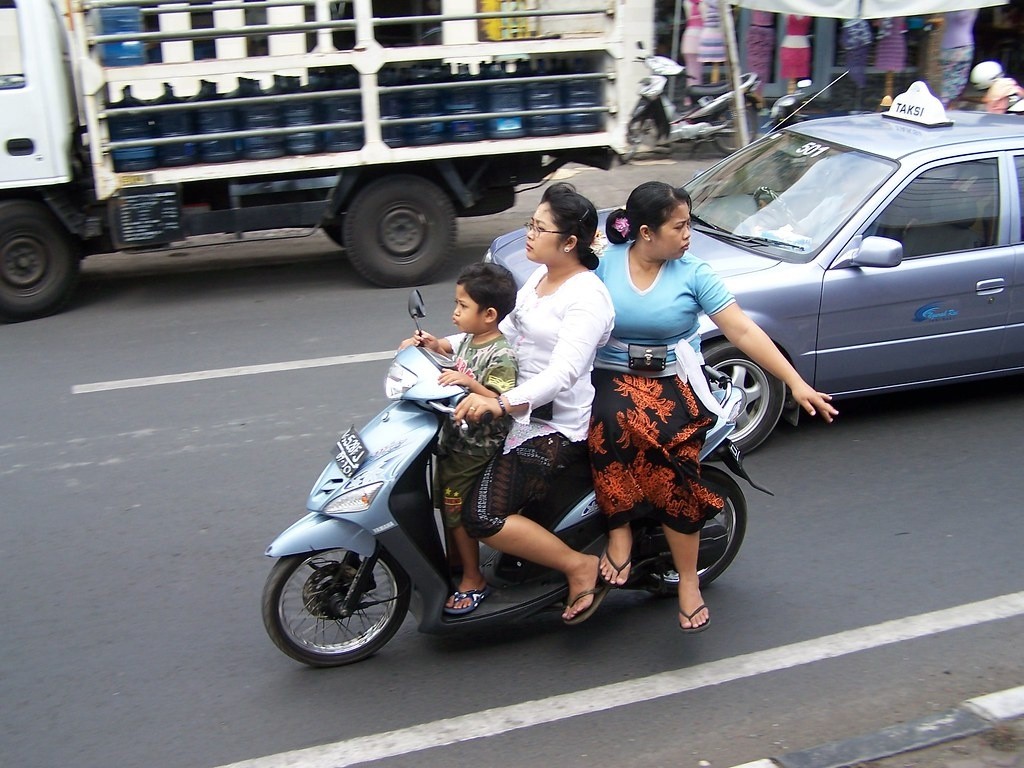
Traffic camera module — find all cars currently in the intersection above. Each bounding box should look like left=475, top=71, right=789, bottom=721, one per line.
left=486, top=77, right=1024, bottom=468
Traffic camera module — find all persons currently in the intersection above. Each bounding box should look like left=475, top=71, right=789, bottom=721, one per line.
left=413, top=263, right=518, bottom=614
left=939, top=9, right=978, bottom=110
left=752, top=161, right=881, bottom=247
left=969, top=61, right=1024, bottom=114
left=584, top=182, right=838, bottom=634
left=394, top=182, right=615, bottom=624
left=681, top=0, right=704, bottom=107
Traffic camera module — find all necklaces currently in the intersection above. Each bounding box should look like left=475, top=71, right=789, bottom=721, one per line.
left=631, top=267, right=657, bottom=290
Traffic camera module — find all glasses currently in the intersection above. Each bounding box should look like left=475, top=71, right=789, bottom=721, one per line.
left=524, top=222, right=571, bottom=237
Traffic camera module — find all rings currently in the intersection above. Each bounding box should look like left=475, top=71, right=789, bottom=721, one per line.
left=469, top=406, right=476, bottom=411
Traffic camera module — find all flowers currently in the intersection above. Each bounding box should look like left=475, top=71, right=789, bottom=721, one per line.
left=611, top=216, right=631, bottom=238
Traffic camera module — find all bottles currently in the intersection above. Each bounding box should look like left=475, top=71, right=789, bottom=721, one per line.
left=151, top=85, right=199, bottom=168
left=109, top=87, right=159, bottom=172
left=187, top=81, right=242, bottom=163
left=225, top=58, right=601, bottom=160
left=97, top=4, right=148, bottom=67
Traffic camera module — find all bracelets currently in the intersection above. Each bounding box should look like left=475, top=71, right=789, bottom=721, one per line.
left=494, top=397, right=506, bottom=417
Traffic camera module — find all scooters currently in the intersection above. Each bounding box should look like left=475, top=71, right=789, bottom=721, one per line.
left=262, top=287, right=779, bottom=671
left=618, top=42, right=767, bottom=166
left=761, top=79, right=860, bottom=132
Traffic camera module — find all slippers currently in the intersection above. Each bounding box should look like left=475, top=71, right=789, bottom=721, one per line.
left=444, top=584, right=491, bottom=614
left=598, top=543, right=631, bottom=588
left=562, top=582, right=613, bottom=623
left=678, top=605, right=712, bottom=634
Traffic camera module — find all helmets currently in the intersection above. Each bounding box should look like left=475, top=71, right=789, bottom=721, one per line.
left=970, top=61, right=1006, bottom=91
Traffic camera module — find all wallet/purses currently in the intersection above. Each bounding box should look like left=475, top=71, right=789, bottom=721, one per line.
left=629, top=343, right=666, bottom=371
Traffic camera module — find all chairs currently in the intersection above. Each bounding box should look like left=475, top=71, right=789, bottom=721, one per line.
left=899, top=188, right=981, bottom=259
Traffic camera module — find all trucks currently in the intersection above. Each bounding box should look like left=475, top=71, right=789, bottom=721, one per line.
left=0, top=0, right=641, bottom=323
